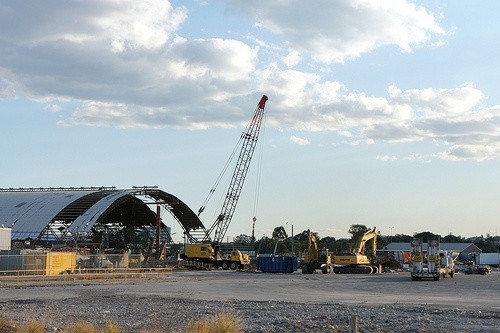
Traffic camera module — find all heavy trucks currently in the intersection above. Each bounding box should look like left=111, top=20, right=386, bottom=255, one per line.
left=408, top=231, right=456, bottom=281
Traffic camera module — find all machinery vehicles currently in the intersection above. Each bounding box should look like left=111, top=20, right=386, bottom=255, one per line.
left=300, top=228, right=332, bottom=274
left=332, top=226, right=380, bottom=275
left=184, top=92, right=269, bottom=269
